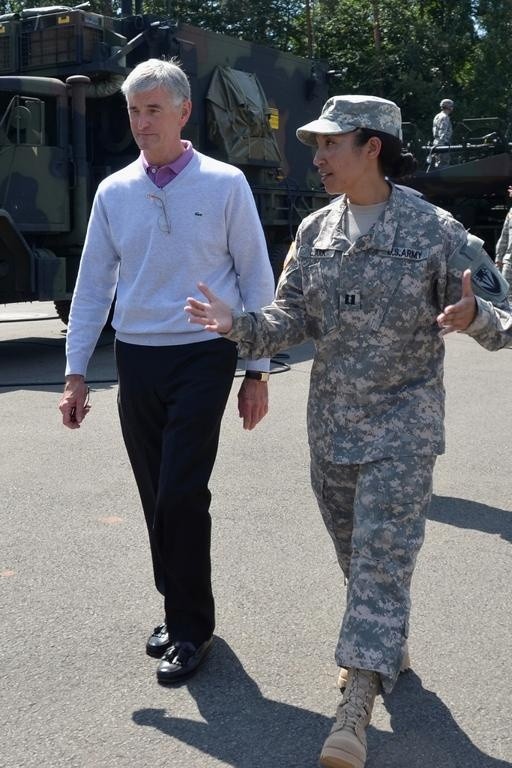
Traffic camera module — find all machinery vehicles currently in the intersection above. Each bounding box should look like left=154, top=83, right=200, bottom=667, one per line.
left=401, top=114, right=510, bottom=251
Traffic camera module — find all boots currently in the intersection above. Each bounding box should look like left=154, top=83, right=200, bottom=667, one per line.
left=337, top=642, right=411, bottom=689
left=319, top=668, right=381, bottom=768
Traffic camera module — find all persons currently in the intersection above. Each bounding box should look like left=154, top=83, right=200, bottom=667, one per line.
left=184, top=94, right=510, bottom=768
left=53, top=55, right=279, bottom=685
left=492, top=186, right=511, bottom=350
left=426, top=97, right=455, bottom=168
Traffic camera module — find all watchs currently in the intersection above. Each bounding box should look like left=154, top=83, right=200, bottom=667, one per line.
left=245, top=370, right=270, bottom=382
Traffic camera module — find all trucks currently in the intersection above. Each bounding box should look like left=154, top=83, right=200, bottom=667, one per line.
left=0, top=0, right=346, bottom=335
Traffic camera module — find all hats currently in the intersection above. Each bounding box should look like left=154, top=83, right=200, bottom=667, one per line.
left=295, top=93, right=404, bottom=148
left=439, top=98, right=454, bottom=109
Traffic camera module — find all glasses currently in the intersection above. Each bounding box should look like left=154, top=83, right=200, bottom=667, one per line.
left=143, top=187, right=173, bottom=237
left=70, top=384, right=93, bottom=421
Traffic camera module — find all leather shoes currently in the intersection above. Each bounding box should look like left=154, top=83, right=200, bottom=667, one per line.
left=145, top=620, right=174, bottom=654
left=156, top=629, right=216, bottom=682
